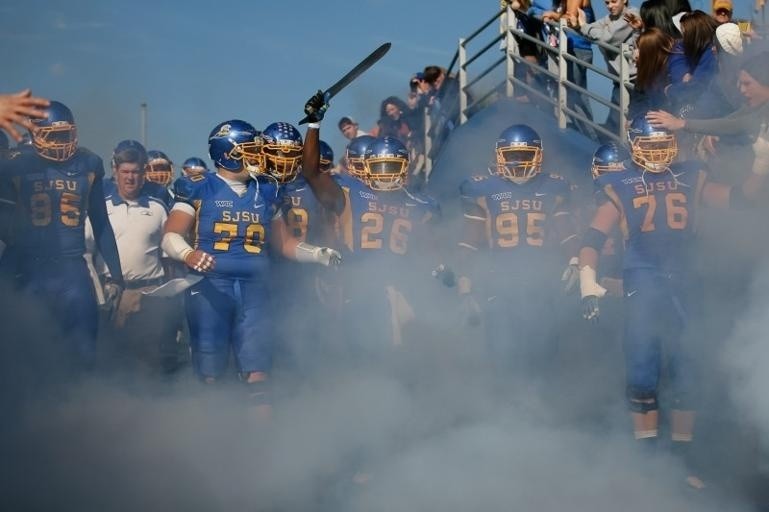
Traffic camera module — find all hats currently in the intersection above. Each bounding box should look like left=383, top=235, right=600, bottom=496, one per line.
left=713, top=0, right=733, bottom=11
left=715, top=22, right=751, bottom=56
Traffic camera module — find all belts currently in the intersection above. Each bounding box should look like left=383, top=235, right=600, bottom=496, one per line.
left=106, top=277, right=164, bottom=290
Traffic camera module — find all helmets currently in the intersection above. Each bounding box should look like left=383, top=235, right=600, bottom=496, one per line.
left=318, top=134, right=409, bottom=192
left=626, top=112, right=678, bottom=174
left=495, top=124, right=544, bottom=184
left=144, top=150, right=173, bottom=186
left=259, top=121, right=304, bottom=183
left=113, top=139, right=148, bottom=169
left=0, top=99, right=79, bottom=161
left=591, top=142, right=630, bottom=181
left=181, top=156, right=207, bottom=177
left=208, top=119, right=267, bottom=177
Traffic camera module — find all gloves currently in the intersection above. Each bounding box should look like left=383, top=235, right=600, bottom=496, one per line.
left=304, top=89, right=331, bottom=123
left=183, top=250, right=217, bottom=273
left=561, top=257, right=580, bottom=296
left=579, top=283, right=615, bottom=327
left=748, top=118, right=769, bottom=176
left=316, top=247, right=342, bottom=271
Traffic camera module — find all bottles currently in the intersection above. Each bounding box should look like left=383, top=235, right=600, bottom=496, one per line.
left=548, top=32, right=558, bottom=48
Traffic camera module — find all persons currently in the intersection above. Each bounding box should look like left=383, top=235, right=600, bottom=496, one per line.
left=1, top=0, right=769, bottom=494
